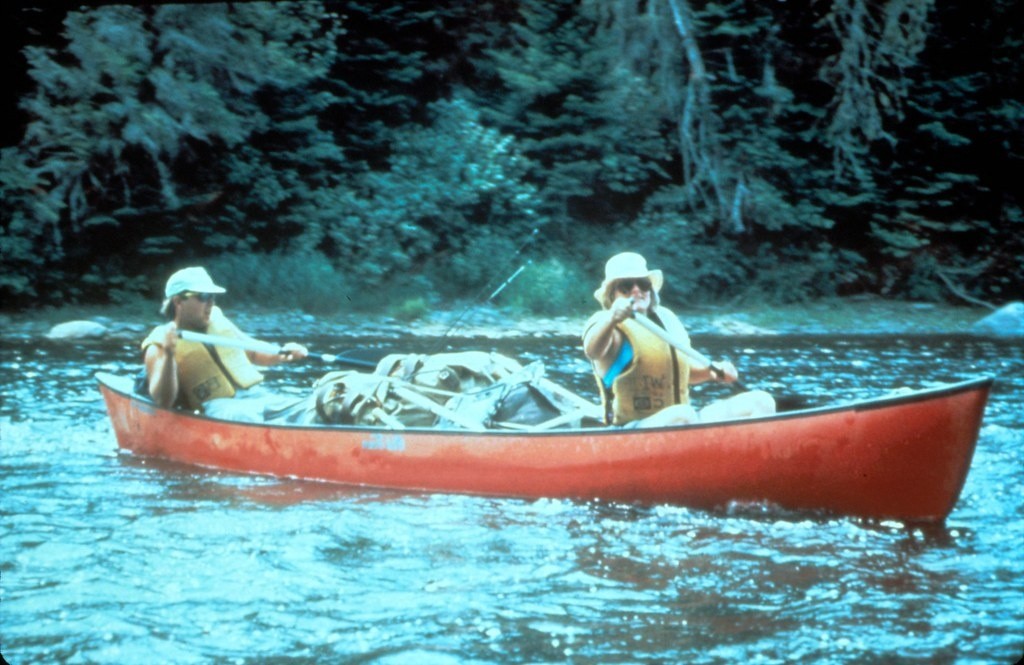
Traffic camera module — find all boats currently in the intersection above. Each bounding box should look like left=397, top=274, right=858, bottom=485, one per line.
left=89, top=367, right=995, bottom=528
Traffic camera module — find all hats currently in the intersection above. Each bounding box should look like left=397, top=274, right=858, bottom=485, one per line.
left=593, top=252, right=664, bottom=308
left=164, top=266, right=226, bottom=297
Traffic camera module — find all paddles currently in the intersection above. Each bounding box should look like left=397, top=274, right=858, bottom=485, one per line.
left=624, top=309, right=810, bottom=407
left=172, top=322, right=376, bottom=368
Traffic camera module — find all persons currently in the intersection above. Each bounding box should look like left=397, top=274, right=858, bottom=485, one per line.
left=143, top=266, right=308, bottom=421
left=584, top=251, right=775, bottom=430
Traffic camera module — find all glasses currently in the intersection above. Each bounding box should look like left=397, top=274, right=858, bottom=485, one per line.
left=183, top=292, right=216, bottom=302
left=614, top=278, right=652, bottom=294
